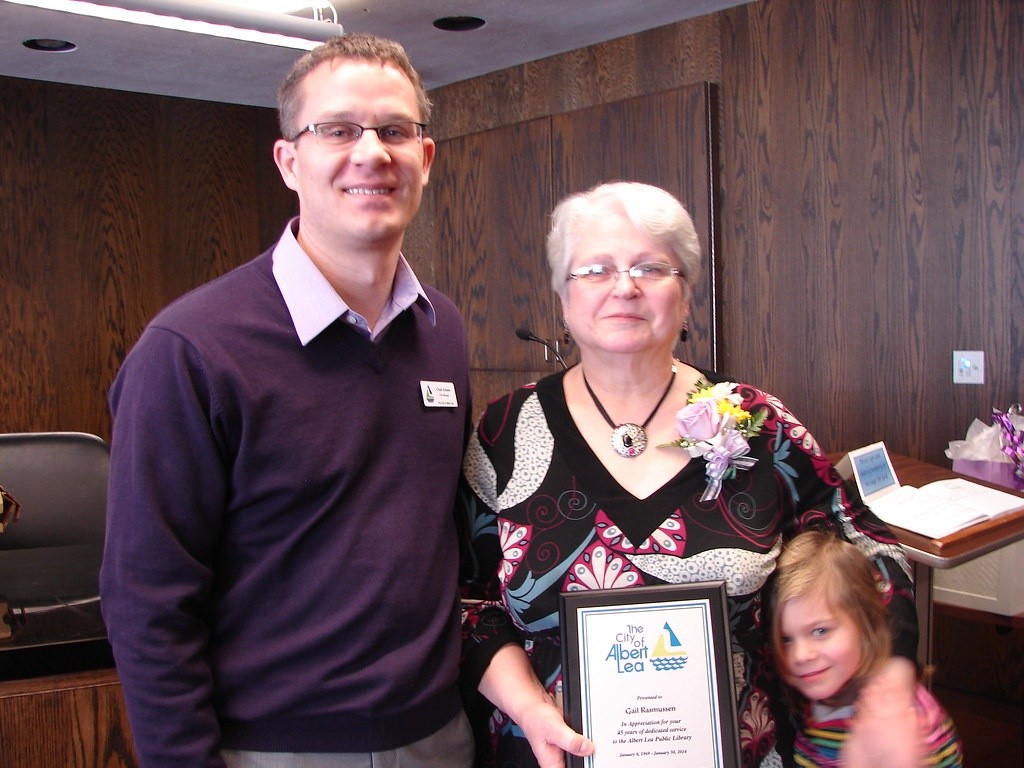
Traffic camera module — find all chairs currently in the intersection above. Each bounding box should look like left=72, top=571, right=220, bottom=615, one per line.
left=1, top=430, right=118, bottom=671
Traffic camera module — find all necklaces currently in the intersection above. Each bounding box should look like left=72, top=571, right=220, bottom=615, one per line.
left=582, top=362, right=677, bottom=457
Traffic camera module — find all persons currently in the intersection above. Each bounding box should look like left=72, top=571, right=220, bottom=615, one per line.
left=100, top=35, right=472, bottom=768
left=773, top=530, right=962, bottom=768
left=458, top=183, right=921, bottom=768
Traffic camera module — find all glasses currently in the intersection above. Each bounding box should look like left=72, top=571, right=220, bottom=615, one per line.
left=285, top=121, right=428, bottom=147
left=564, top=259, right=687, bottom=284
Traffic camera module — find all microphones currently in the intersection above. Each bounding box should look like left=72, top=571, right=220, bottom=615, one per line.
left=516, top=329, right=568, bottom=370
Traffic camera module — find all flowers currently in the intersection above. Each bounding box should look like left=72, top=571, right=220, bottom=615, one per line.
left=658, top=380, right=767, bottom=502
